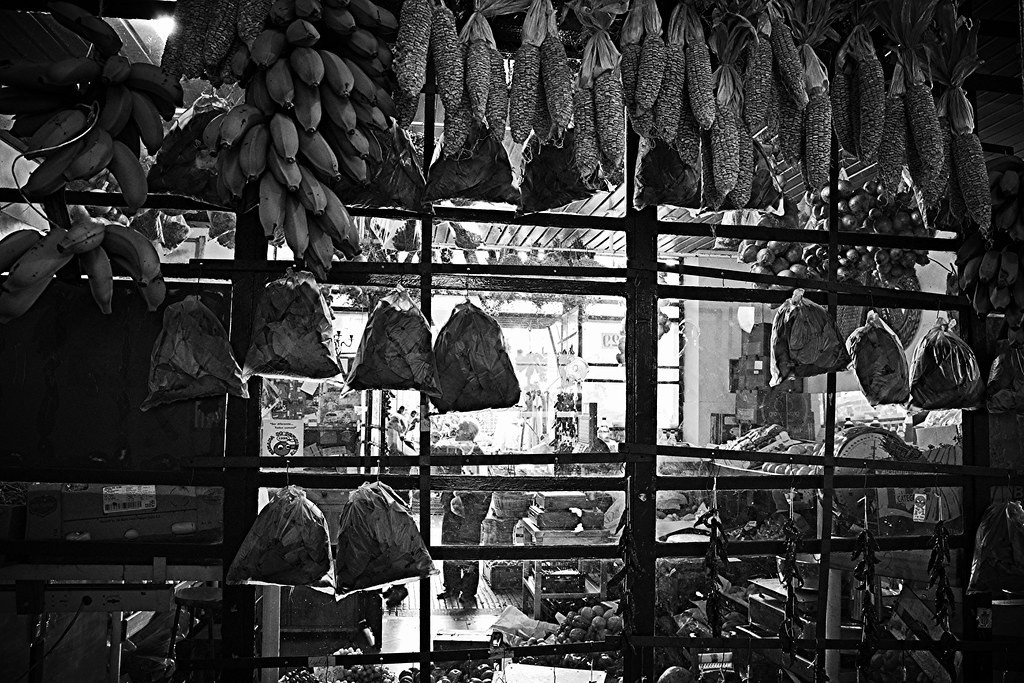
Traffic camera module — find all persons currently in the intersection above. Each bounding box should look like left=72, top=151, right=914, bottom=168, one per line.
left=378, top=411, right=416, bottom=607
left=525, top=391, right=533, bottom=412
left=427, top=420, right=493, bottom=603
left=395, top=406, right=406, bottom=417
left=531, top=389, right=544, bottom=414
left=536, top=389, right=547, bottom=411
left=407, top=410, right=421, bottom=430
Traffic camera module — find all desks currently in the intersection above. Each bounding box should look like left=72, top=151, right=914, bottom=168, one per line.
left=520, top=517, right=612, bottom=622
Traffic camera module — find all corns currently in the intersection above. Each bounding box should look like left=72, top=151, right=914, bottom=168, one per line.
left=394, top=1, right=991, bottom=236
left=160, top=0, right=279, bottom=85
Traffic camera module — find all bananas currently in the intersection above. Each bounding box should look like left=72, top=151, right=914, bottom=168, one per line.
left=956, top=169, right=1023, bottom=348
left=204, top=0, right=402, bottom=272
left=0, top=56, right=184, bottom=324
